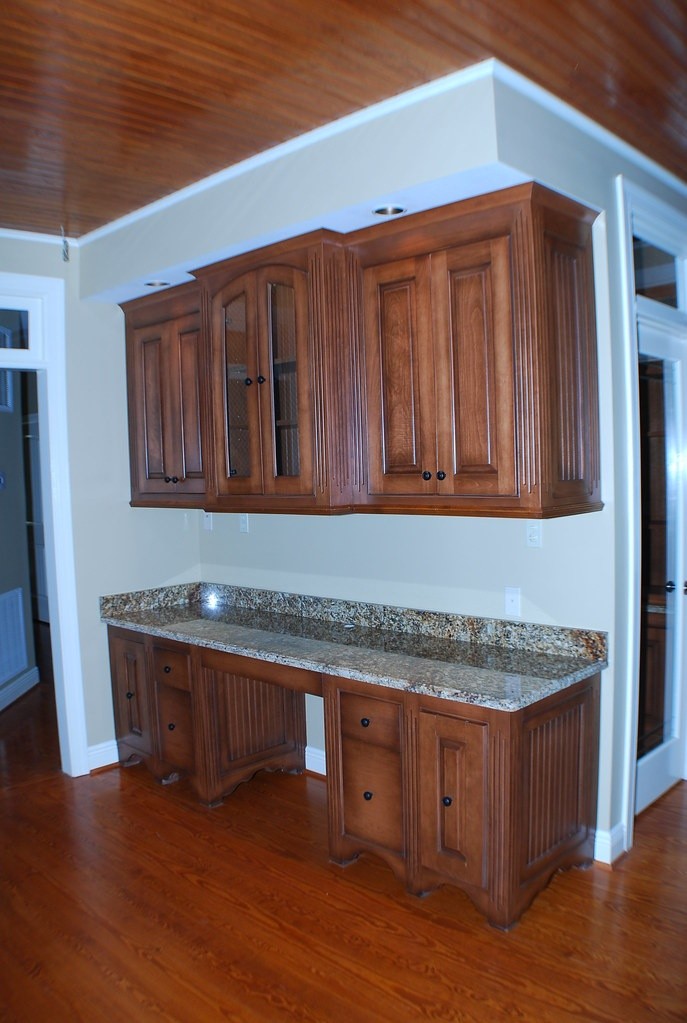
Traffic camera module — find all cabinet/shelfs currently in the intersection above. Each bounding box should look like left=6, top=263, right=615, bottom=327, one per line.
left=324, top=674, right=602, bottom=930
left=117, top=179, right=605, bottom=518
left=637, top=362, right=668, bottom=759
left=107, top=623, right=309, bottom=808
